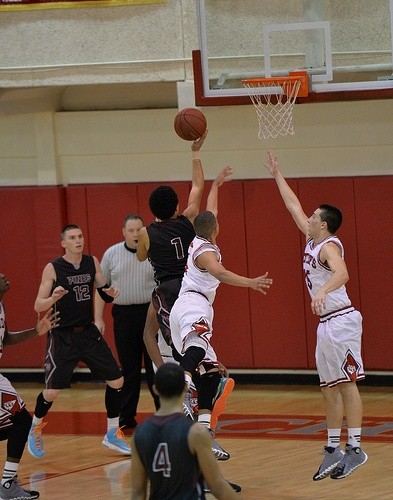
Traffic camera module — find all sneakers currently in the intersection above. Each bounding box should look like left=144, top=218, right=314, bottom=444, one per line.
left=211, top=437, right=230, bottom=460
left=330, top=443, right=368, bottom=479
left=102, top=427, right=132, bottom=455
left=182, top=392, right=195, bottom=421
left=313, top=446, right=346, bottom=481
left=211, top=378, right=235, bottom=418
left=0, top=474, right=39, bottom=500
left=27, top=422, right=48, bottom=458
left=204, top=479, right=241, bottom=493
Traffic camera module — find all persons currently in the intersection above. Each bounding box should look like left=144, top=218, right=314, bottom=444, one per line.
left=129, top=363, right=245, bottom=500
left=264, top=148, right=368, bottom=482
left=94, top=215, right=158, bottom=438
left=135, top=128, right=235, bottom=430
left=26, top=224, right=133, bottom=458
left=0, top=271, right=61, bottom=500
left=169, top=166, right=273, bottom=460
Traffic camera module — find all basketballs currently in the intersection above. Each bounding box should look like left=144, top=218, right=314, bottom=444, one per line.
left=174, top=108, right=207, bottom=141
